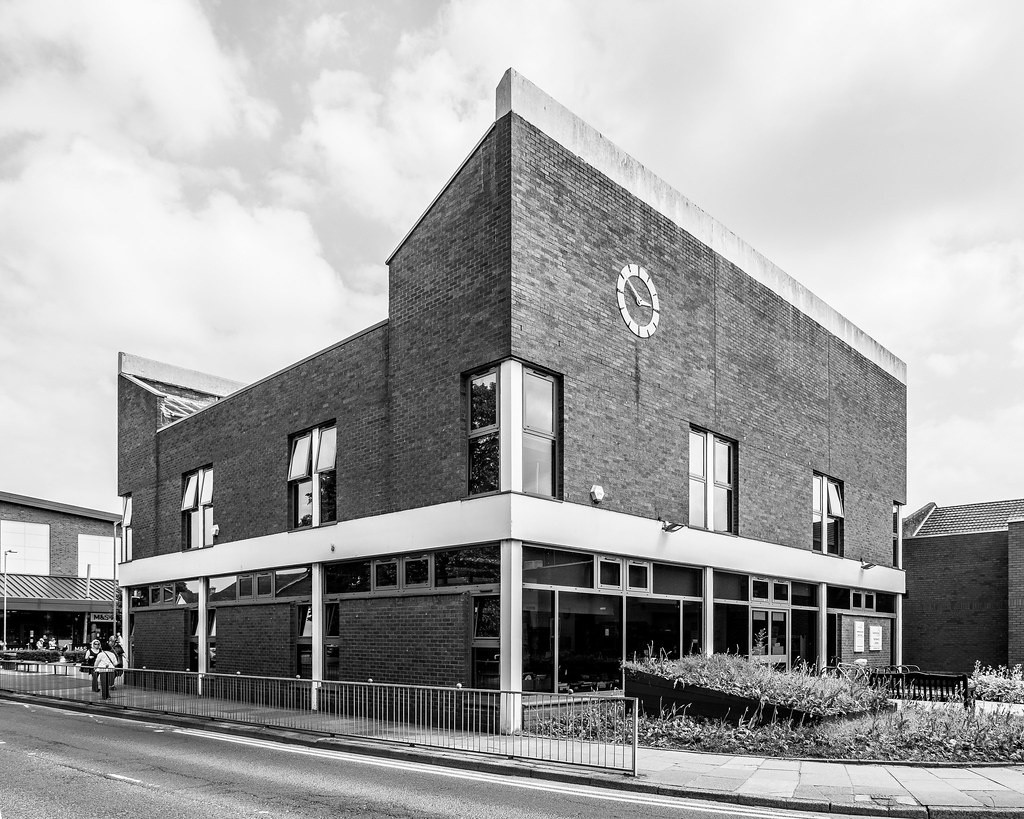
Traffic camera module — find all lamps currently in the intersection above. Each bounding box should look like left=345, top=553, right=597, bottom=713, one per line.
left=662, top=521, right=684, bottom=532
left=860, top=558, right=876, bottom=569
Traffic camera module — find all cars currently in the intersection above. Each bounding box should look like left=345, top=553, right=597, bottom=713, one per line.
left=326, top=646, right=339, bottom=666
left=194, top=648, right=217, bottom=666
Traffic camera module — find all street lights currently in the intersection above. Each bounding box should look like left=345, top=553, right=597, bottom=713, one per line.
left=4, top=549, right=19, bottom=653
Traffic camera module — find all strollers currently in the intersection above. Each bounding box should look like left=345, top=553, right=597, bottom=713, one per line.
left=55, top=645, right=68, bottom=656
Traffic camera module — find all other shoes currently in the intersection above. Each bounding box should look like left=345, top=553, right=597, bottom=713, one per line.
left=94, top=689, right=100, bottom=692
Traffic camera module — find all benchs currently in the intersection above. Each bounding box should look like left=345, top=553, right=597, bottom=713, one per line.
left=868, top=670, right=974, bottom=711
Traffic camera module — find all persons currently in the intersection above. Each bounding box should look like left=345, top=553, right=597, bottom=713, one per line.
left=94, top=642, right=119, bottom=700
left=558, top=663, right=570, bottom=692
left=14, top=636, right=22, bottom=645
left=28, top=630, right=38, bottom=650
left=37, top=634, right=49, bottom=650
left=49, top=636, right=57, bottom=650
left=85, top=639, right=102, bottom=692
left=109, top=635, right=129, bottom=689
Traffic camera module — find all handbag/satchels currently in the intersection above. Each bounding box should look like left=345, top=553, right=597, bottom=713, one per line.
left=115, top=664, right=122, bottom=676
left=79, top=657, right=90, bottom=672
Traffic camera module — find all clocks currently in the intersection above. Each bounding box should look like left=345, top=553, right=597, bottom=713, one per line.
left=616, top=263, right=660, bottom=338
